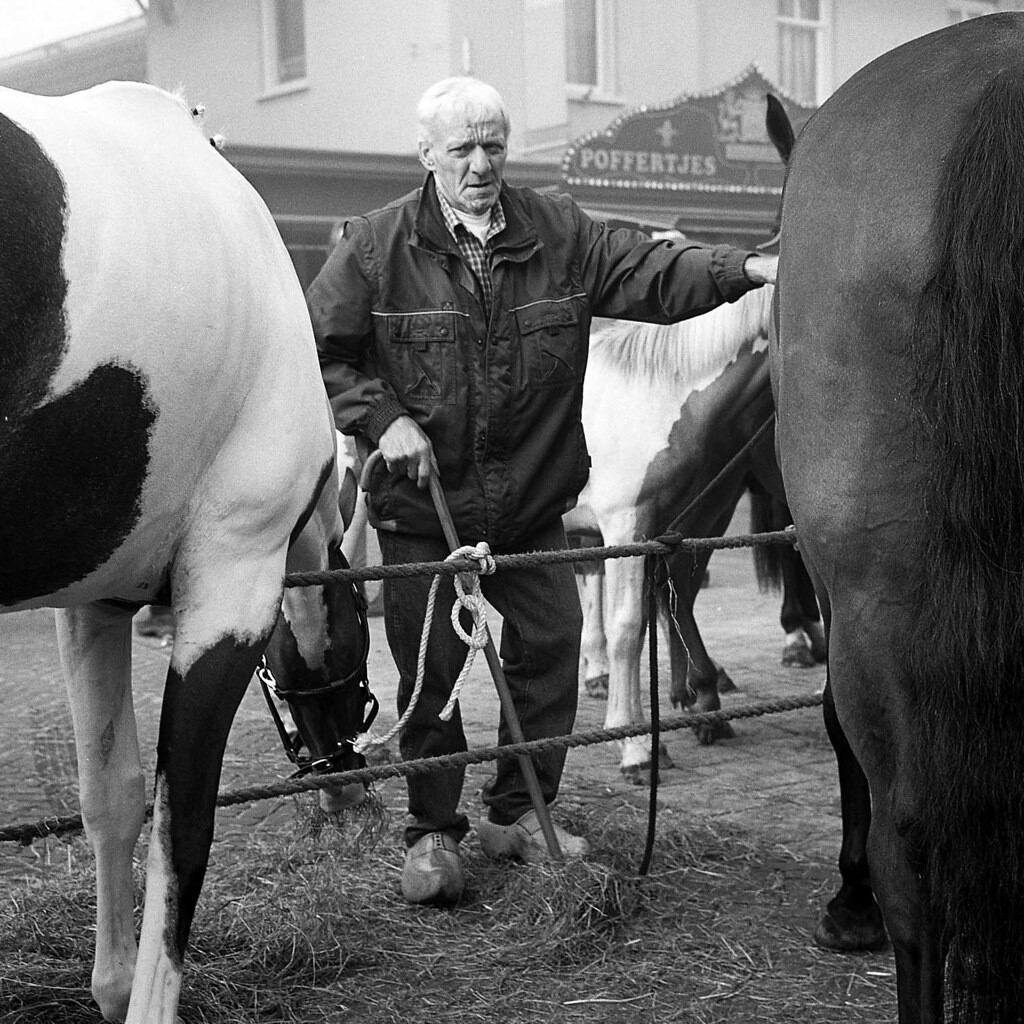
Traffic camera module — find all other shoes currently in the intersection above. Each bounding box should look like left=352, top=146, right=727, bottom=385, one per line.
left=402, top=831, right=467, bottom=901
left=477, top=808, right=590, bottom=866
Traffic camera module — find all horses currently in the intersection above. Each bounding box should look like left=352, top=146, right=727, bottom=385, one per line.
left=0, top=77, right=385, bottom=1024
left=336, top=12, right=1021, bottom=1023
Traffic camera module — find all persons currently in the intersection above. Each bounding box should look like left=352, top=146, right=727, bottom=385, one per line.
left=308, top=76, right=783, bottom=902
